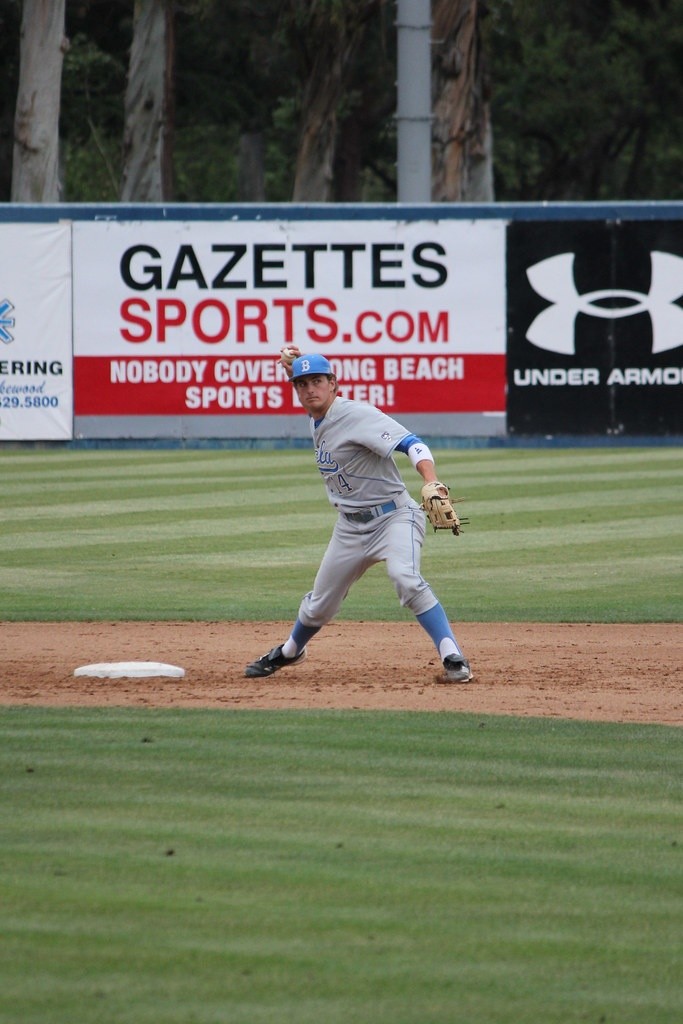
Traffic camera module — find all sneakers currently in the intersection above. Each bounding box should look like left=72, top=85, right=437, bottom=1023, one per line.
left=443, top=653, right=473, bottom=683
left=244, top=644, right=307, bottom=678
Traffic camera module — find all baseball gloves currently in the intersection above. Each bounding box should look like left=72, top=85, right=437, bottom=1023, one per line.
left=417, top=482, right=474, bottom=537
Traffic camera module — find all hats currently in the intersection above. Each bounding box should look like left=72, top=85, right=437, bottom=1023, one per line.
left=288, top=354, right=333, bottom=380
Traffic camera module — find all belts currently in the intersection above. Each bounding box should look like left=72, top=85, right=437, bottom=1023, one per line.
left=345, top=500, right=396, bottom=522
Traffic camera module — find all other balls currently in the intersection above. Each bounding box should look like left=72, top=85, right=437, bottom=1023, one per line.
left=281, top=348, right=297, bottom=364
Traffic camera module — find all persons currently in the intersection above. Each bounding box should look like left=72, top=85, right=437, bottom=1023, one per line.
left=245, top=345, right=475, bottom=683
